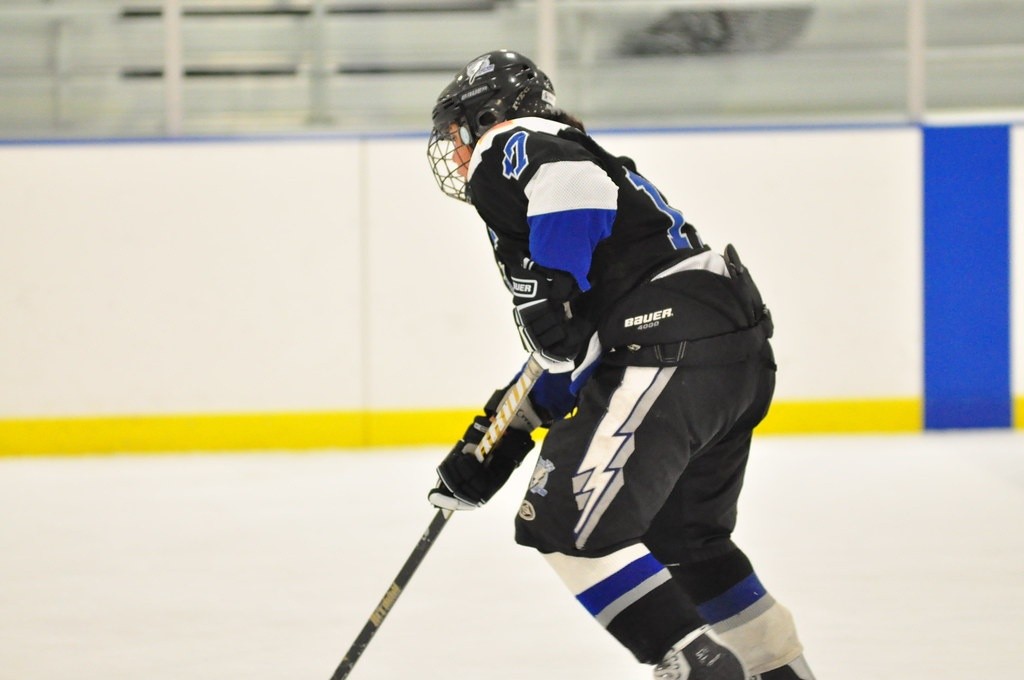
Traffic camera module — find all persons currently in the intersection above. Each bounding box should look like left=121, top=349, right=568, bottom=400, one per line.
left=427, top=50, right=818, bottom=680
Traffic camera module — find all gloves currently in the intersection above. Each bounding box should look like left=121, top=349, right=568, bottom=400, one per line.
left=427, top=416, right=536, bottom=512
left=512, top=258, right=589, bottom=374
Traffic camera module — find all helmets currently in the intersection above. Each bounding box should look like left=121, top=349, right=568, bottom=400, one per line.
left=428, top=48, right=556, bottom=205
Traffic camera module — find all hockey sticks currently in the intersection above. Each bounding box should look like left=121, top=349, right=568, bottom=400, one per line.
left=329, top=354, right=545, bottom=680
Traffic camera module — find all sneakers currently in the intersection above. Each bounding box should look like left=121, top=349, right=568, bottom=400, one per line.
left=748, top=655, right=816, bottom=680
left=654, top=624, right=749, bottom=680
